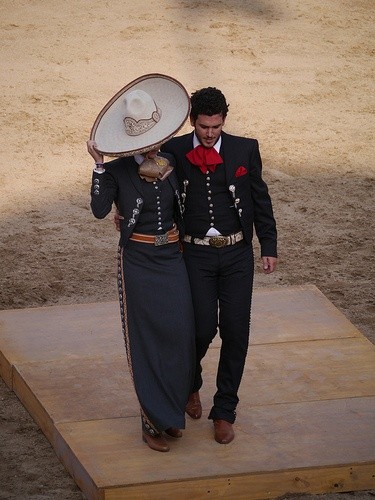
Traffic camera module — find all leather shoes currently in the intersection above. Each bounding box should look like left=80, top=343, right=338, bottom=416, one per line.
left=184, top=390, right=202, bottom=420
left=165, top=427, right=182, bottom=438
left=212, top=419, right=235, bottom=445
left=141, top=429, right=170, bottom=453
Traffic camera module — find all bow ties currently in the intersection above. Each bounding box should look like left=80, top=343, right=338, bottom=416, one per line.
left=185, top=144, right=223, bottom=174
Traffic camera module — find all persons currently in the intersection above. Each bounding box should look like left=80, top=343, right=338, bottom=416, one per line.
left=87, top=139, right=202, bottom=452
left=114, top=86, right=279, bottom=444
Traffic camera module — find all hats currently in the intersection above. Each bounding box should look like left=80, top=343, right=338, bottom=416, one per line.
left=88, top=73, right=192, bottom=159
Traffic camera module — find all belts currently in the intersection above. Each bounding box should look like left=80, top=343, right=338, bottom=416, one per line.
left=183, top=232, right=243, bottom=248
left=128, top=225, right=179, bottom=246
